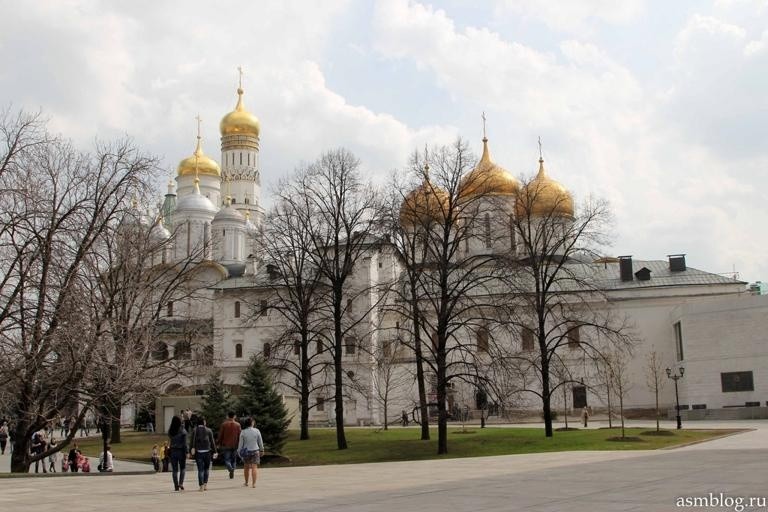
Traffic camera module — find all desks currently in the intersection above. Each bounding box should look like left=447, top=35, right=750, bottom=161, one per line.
left=665, top=366, right=684, bottom=428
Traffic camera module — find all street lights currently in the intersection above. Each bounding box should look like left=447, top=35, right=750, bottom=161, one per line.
left=165, top=447, right=170, bottom=457
left=240, top=448, right=248, bottom=457
left=189, top=448, right=195, bottom=459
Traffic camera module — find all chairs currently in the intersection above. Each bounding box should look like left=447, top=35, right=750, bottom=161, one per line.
left=178, top=484, right=184, bottom=489
left=175, top=487, right=178, bottom=491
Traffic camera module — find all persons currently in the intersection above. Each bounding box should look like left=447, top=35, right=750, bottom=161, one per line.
left=61, top=452, right=70, bottom=471
left=180, top=409, right=187, bottom=426
left=214, top=411, right=242, bottom=479
left=159, top=440, right=171, bottom=471
left=75, top=451, right=84, bottom=468
left=82, top=457, right=89, bottom=472
left=136, top=415, right=143, bottom=429
left=68, top=442, right=82, bottom=472
left=151, top=444, right=160, bottom=470
left=184, top=407, right=193, bottom=419
left=401, top=409, right=409, bottom=425
left=236, top=417, right=266, bottom=489
left=145, top=415, right=155, bottom=433
left=187, top=416, right=219, bottom=491
left=1, top=413, right=101, bottom=455
left=45, top=438, right=58, bottom=472
left=167, top=415, right=192, bottom=491
left=99, top=444, right=114, bottom=472
left=33, top=434, right=47, bottom=474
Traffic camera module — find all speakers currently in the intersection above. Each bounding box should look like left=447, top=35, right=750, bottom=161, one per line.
left=198, top=483, right=206, bottom=491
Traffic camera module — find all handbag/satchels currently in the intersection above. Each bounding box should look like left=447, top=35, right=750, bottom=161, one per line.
left=260, top=450, right=265, bottom=452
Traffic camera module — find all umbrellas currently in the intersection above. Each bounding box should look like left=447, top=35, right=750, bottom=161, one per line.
left=230, top=469, right=234, bottom=478
